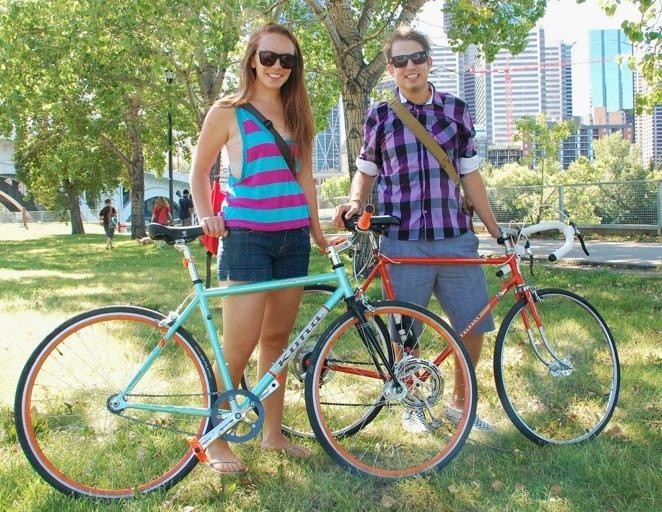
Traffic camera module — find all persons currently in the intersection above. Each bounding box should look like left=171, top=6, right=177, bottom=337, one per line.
left=179, top=189, right=192, bottom=226
left=176, top=190, right=181, bottom=198
left=22, top=207, right=26, bottom=226
left=99, top=199, right=117, bottom=249
left=151, top=196, right=174, bottom=249
left=331, top=21, right=518, bottom=434
left=189, top=23, right=329, bottom=474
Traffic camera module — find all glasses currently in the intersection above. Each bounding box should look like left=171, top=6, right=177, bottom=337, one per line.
left=390, top=51, right=428, bottom=68
left=256, top=51, right=296, bottom=69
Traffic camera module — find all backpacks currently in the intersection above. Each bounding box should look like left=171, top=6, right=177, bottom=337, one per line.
left=108, top=205, right=118, bottom=229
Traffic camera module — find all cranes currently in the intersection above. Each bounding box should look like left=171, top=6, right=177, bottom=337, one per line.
left=464, top=51, right=614, bottom=164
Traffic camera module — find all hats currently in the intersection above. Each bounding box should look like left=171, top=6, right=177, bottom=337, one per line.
left=183, top=189, right=189, bottom=194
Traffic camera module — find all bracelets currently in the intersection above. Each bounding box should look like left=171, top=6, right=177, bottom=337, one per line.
left=351, top=198, right=361, bottom=209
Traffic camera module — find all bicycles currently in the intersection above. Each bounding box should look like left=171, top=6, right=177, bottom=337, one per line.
left=236, top=200, right=623, bottom=449
left=13, top=203, right=484, bottom=505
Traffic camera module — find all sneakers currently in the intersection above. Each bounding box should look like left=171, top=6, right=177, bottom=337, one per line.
left=444, top=400, right=493, bottom=434
left=402, top=407, right=433, bottom=434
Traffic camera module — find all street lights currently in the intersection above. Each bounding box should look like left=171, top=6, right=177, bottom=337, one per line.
left=161, top=64, right=177, bottom=216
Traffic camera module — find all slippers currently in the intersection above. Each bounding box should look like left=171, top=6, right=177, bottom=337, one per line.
left=206, top=456, right=249, bottom=475
left=285, top=445, right=311, bottom=458
